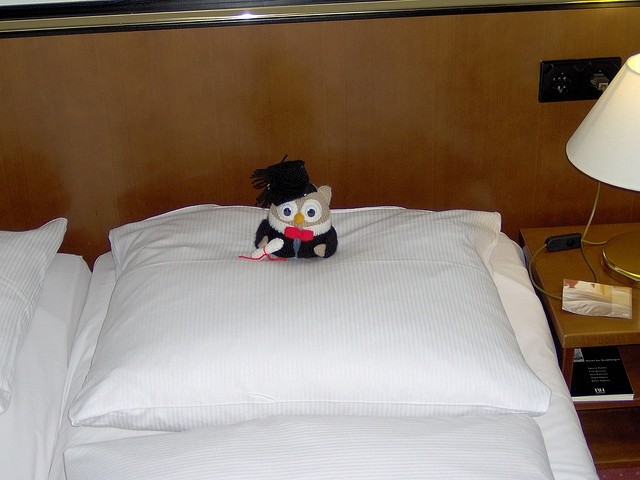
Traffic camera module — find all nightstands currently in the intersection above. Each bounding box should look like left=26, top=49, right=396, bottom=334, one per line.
left=518, top=223, right=640, bottom=469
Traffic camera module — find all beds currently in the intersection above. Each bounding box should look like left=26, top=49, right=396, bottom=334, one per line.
left=0, top=0, right=640, bottom=478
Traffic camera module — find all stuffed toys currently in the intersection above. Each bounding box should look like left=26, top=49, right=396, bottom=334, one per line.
left=238, top=154, right=338, bottom=262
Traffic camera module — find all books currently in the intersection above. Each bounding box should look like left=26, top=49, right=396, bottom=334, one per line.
left=570, top=347, right=635, bottom=402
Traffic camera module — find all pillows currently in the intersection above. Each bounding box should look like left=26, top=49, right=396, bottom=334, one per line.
left=0, top=216, right=68, bottom=416
left=66, top=204, right=551, bottom=431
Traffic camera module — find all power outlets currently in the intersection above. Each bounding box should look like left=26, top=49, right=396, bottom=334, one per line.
left=538, top=56, right=621, bottom=102
